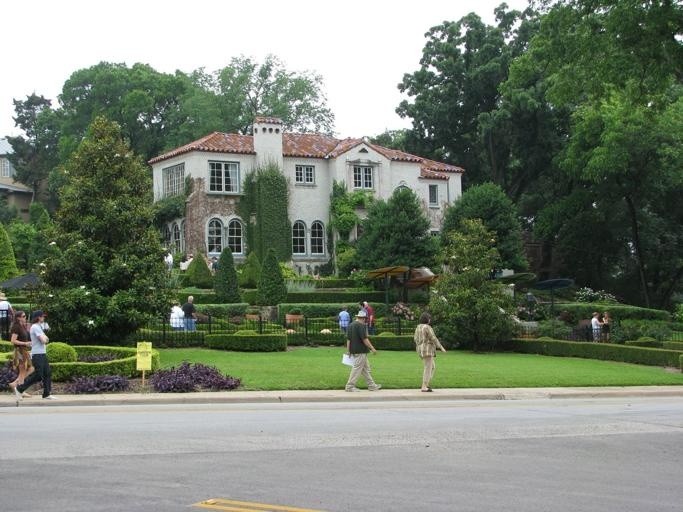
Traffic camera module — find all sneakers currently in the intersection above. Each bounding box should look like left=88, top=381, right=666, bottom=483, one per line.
left=367, top=383, right=381, bottom=390
left=9, top=382, right=55, bottom=401
left=345, top=387, right=359, bottom=392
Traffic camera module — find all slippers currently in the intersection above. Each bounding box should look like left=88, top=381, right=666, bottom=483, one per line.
left=422, top=388, right=433, bottom=392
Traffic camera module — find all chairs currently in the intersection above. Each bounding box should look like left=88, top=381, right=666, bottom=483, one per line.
left=0, top=309, right=8, bottom=340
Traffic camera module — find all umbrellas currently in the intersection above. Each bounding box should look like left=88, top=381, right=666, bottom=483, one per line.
left=500, top=269, right=575, bottom=321
left=367, top=264, right=442, bottom=297
left=0, top=272, right=42, bottom=314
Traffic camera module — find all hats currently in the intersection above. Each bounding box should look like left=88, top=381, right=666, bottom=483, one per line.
left=31, top=310, right=47, bottom=319
left=0, top=293, right=7, bottom=300
left=354, top=311, right=368, bottom=319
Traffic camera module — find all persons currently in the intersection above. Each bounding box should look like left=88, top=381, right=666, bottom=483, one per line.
left=0, top=293, right=56, bottom=399
left=168, top=296, right=198, bottom=333
left=414, top=313, right=445, bottom=392
left=346, top=310, right=384, bottom=391
left=162, top=248, right=224, bottom=281
left=338, top=300, right=375, bottom=332
left=591, top=311, right=610, bottom=341
